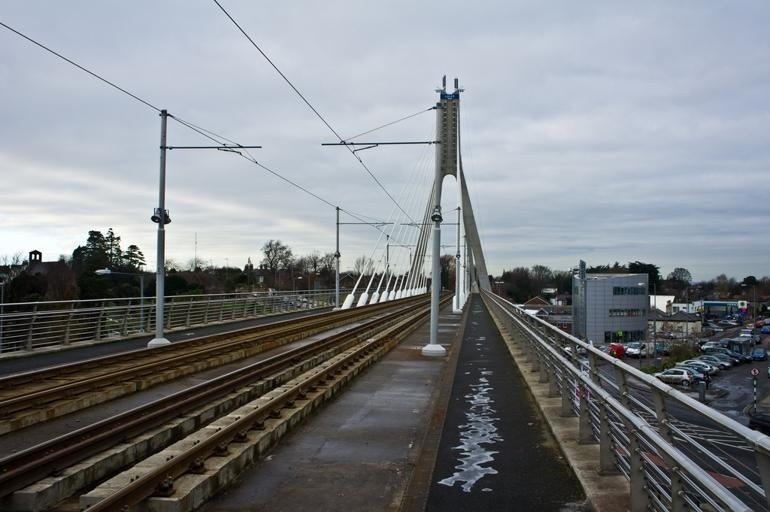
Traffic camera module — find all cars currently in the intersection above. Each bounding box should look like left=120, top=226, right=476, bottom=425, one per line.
left=564, top=340, right=593, bottom=355
left=596, top=317, right=769, bottom=387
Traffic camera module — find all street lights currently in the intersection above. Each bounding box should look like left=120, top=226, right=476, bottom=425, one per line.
left=94, top=269, right=143, bottom=332
left=294, top=276, right=302, bottom=295
